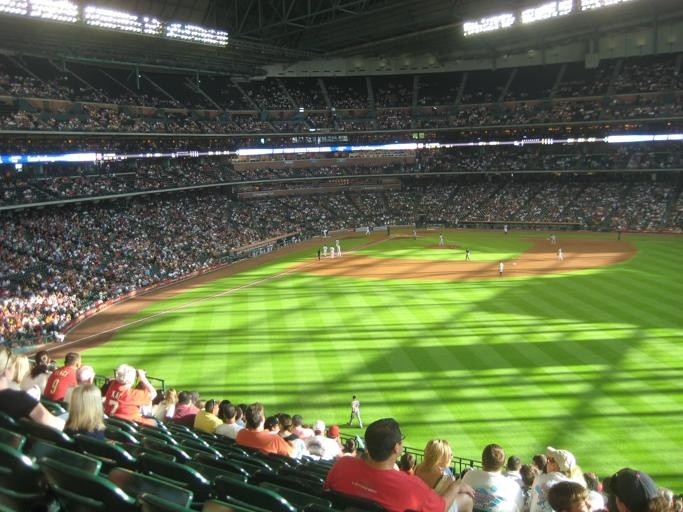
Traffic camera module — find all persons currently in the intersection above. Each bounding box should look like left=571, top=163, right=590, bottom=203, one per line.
left=1, top=55, right=312, bottom=346
left=0, top=346, right=683, bottom=512
left=316, top=225, right=565, bottom=276
left=226, top=54, right=681, bottom=239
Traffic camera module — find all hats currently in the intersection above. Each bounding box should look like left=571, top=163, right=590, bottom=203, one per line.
left=328, top=425, right=339, bottom=437
left=314, top=420, right=324, bottom=431
left=76, top=365, right=95, bottom=381
left=612, top=468, right=658, bottom=503
left=547, top=446, right=576, bottom=471
left=114, top=364, right=136, bottom=384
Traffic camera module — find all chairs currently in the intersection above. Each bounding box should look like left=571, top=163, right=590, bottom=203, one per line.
left=0, top=398, right=405, bottom=512
left=0, top=47, right=682, bottom=113
